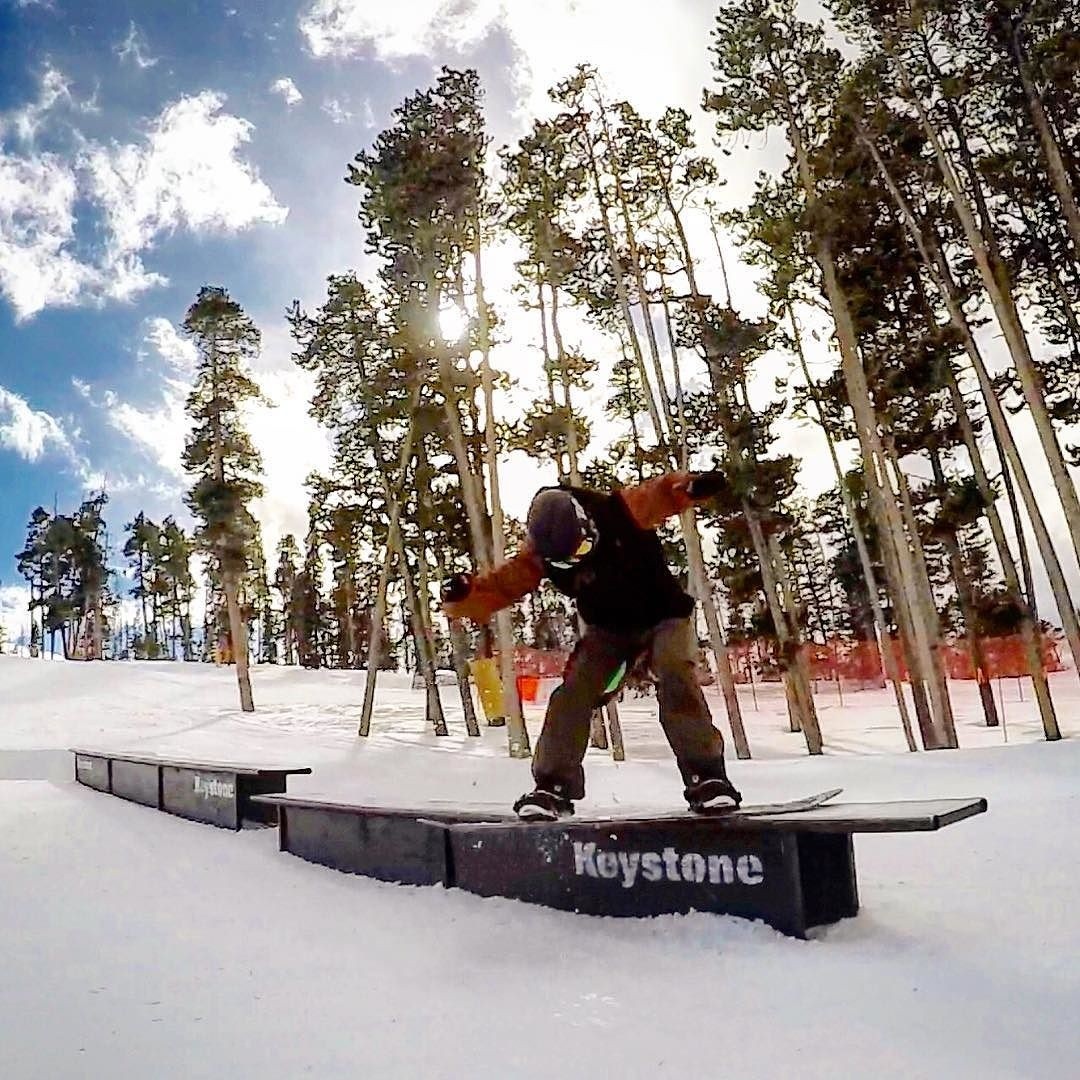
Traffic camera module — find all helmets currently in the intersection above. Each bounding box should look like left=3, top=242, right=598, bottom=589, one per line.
left=529, top=489, right=581, bottom=560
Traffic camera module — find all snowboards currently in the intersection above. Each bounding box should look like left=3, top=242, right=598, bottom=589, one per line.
left=417, top=787, right=845, bottom=831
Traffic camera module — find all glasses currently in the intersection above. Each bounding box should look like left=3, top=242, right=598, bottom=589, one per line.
left=550, top=537, right=594, bottom=570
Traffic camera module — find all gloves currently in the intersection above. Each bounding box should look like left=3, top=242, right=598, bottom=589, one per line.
left=440, top=573, right=473, bottom=603
left=692, top=474, right=727, bottom=497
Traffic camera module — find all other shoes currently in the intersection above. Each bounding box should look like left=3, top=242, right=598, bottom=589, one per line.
left=514, top=786, right=575, bottom=821
left=692, top=782, right=742, bottom=815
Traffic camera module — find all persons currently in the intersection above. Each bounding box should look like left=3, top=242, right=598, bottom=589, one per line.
left=441, top=470, right=744, bottom=823
